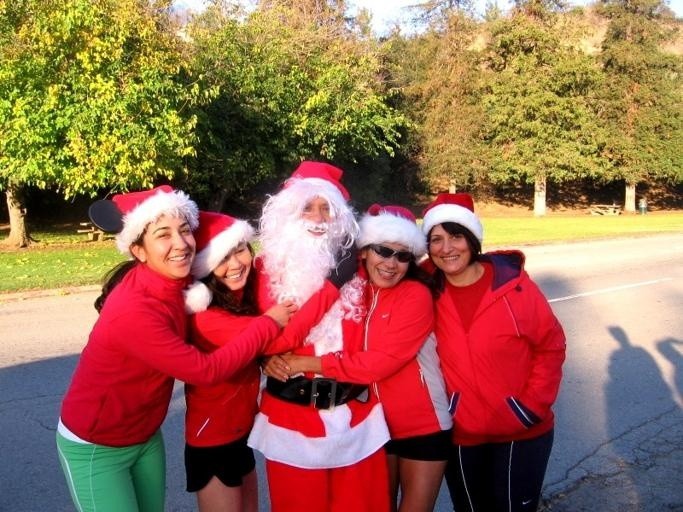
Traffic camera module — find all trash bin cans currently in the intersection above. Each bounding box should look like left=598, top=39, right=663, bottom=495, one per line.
left=639, top=199, right=647, bottom=215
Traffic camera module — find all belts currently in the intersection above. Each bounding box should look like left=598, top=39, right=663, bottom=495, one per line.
left=266, top=377, right=368, bottom=409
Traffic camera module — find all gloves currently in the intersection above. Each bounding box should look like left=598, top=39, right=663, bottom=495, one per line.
left=326, top=234, right=360, bottom=289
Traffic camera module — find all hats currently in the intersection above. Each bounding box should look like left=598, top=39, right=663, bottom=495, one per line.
left=354, top=204, right=427, bottom=264
left=110, top=185, right=199, bottom=257
left=182, top=208, right=257, bottom=314
left=422, top=192, right=483, bottom=256
left=279, top=161, right=351, bottom=207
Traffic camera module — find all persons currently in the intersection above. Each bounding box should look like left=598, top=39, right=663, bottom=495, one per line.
left=54, top=184, right=298, bottom=511
left=245, top=160, right=394, bottom=511
left=263, top=203, right=454, bottom=512
left=421, top=194, right=567, bottom=510
left=183, top=210, right=358, bottom=512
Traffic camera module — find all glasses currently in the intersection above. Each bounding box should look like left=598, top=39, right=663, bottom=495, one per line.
left=367, top=243, right=413, bottom=262
